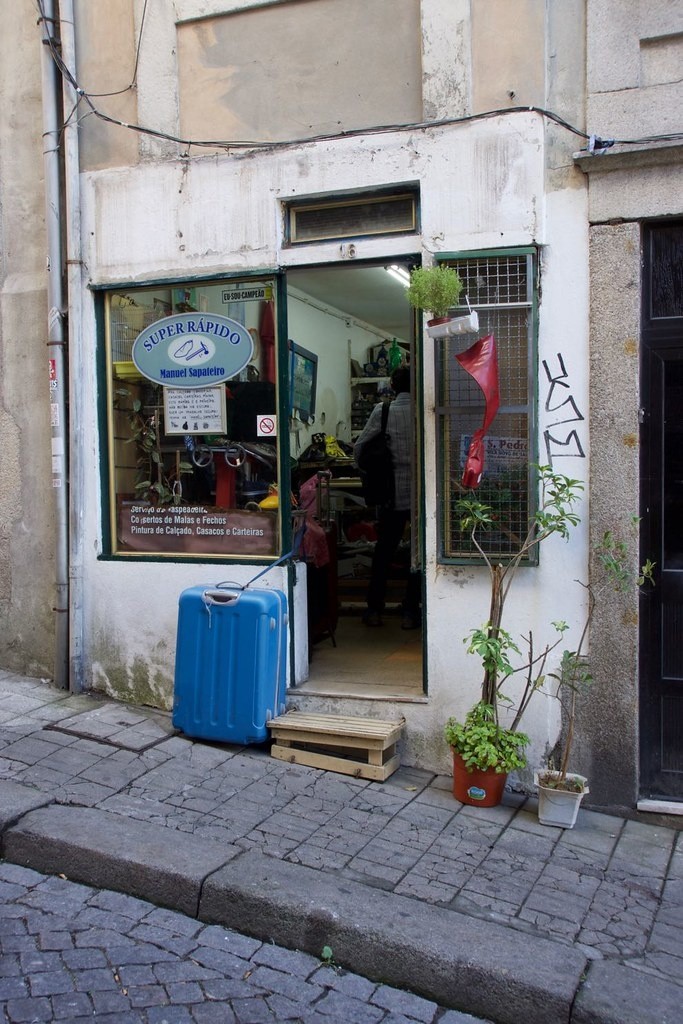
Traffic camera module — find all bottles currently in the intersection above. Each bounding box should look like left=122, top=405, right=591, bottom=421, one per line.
left=376, top=342, right=388, bottom=377
left=388, top=337, right=402, bottom=377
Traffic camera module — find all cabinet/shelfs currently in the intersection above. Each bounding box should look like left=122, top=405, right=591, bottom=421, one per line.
left=346, top=339, right=391, bottom=444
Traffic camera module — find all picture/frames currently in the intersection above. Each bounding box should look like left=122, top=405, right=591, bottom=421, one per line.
left=288, top=342, right=319, bottom=423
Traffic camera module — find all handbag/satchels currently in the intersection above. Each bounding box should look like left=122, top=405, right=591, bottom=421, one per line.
left=292, top=516, right=328, bottom=585
left=296, top=433, right=355, bottom=462
left=361, top=400, right=396, bottom=509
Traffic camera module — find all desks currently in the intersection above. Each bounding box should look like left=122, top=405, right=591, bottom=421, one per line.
left=160, top=444, right=243, bottom=509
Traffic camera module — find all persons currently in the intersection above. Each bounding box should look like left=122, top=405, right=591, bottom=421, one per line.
left=353, top=366, right=420, bottom=631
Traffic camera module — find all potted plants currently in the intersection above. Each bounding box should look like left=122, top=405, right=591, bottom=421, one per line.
left=532, top=515, right=657, bottom=830
left=401, top=263, right=464, bottom=327
left=443, top=460, right=586, bottom=809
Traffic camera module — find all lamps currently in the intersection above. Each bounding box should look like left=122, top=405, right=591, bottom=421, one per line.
left=384, top=264, right=410, bottom=288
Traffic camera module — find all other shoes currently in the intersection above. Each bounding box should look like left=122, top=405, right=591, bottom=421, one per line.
left=362, top=610, right=381, bottom=627
left=401, top=615, right=421, bottom=628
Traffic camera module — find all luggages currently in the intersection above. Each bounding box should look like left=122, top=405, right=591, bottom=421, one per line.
left=302, top=471, right=339, bottom=648
left=173, top=580, right=288, bottom=744
